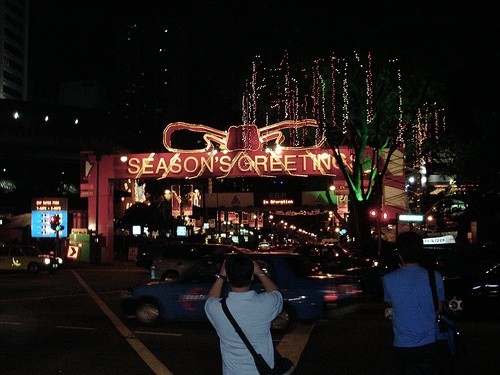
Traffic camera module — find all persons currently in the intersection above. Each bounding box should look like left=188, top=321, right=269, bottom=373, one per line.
left=204, top=252, right=283, bottom=375
left=382, top=231, right=445, bottom=375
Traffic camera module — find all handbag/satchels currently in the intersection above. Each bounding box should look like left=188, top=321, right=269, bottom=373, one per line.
left=255, top=350, right=293, bottom=375
left=438, top=312, right=456, bottom=354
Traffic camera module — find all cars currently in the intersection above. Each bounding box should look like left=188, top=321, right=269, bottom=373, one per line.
left=134, top=233, right=500, bottom=323
left=150, top=239, right=248, bottom=282
left=109, top=250, right=367, bottom=337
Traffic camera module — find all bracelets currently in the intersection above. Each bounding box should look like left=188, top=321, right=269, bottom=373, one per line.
left=215, top=273, right=226, bottom=281
left=259, top=268, right=267, bottom=277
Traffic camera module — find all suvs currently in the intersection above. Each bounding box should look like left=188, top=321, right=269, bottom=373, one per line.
left=0, top=244, right=59, bottom=274
